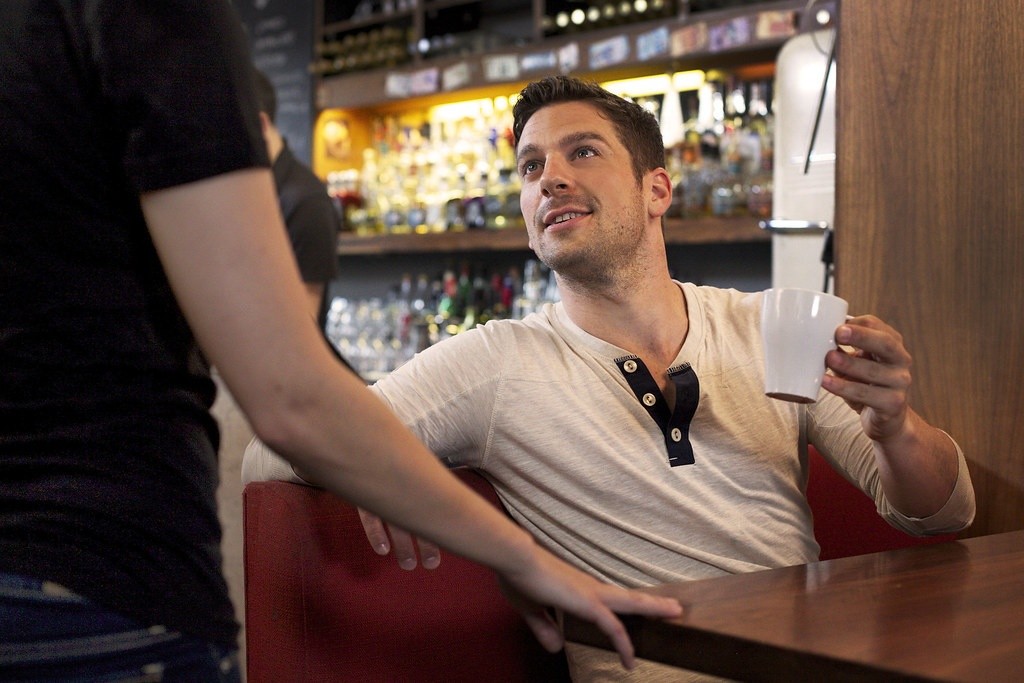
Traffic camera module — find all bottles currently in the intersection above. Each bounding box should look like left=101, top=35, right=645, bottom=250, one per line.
left=638, top=78, right=773, bottom=215
left=322, top=258, right=559, bottom=386
left=324, top=118, right=526, bottom=233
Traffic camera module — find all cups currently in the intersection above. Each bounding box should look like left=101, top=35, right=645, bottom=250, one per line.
left=761, top=288, right=855, bottom=404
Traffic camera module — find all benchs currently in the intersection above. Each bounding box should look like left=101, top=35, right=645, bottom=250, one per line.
left=240, top=441, right=1024, bottom=683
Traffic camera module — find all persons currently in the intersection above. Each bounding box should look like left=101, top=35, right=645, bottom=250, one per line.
left=241, top=75, right=976, bottom=683
left=248, top=65, right=338, bottom=333
left=0, top=0, right=683, bottom=683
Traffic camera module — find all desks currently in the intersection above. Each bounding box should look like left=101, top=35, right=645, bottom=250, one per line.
left=564, top=529, right=1024, bottom=683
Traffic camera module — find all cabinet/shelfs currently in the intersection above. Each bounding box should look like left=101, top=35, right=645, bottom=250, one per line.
left=312, top=46, right=776, bottom=383
